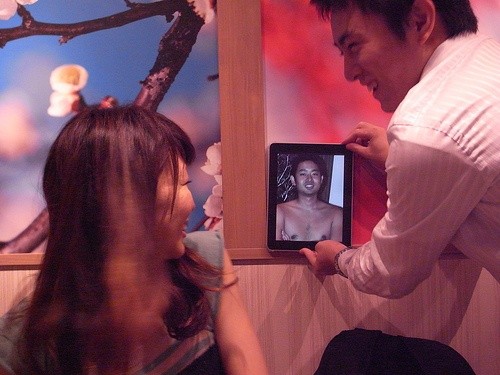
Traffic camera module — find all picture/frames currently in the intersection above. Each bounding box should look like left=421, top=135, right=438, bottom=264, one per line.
left=265, top=141, right=354, bottom=254
left=0, top=0, right=275, bottom=269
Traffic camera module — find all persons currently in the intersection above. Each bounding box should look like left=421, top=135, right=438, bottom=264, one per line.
left=298, top=0, right=500, bottom=285
left=275, top=156, right=343, bottom=241
left=0, top=104, right=272, bottom=375
